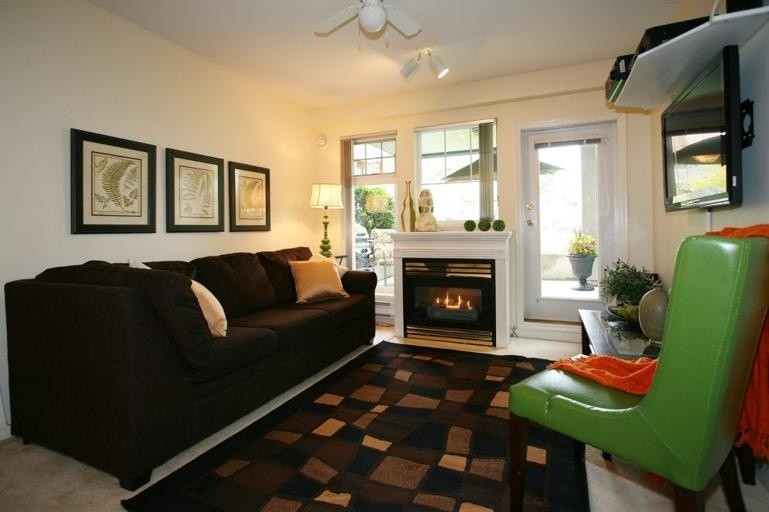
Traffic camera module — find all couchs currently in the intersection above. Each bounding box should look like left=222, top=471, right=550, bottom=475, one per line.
left=4, top=245, right=378, bottom=491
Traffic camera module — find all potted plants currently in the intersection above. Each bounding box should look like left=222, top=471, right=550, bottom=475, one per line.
left=601, top=261, right=662, bottom=325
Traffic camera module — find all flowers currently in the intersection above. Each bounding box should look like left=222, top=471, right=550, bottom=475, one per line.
left=565, top=232, right=600, bottom=256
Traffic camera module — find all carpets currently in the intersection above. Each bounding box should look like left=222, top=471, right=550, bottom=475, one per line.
left=120, top=340, right=591, bottom=511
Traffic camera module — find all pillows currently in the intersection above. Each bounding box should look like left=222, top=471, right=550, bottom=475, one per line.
left=307, top=252, right=350, bottom=280
left=128, top=261, right=152, bottom=269
left=189, top=276, right=228, bottom=338
left=288, top=259, right=351, bottom=304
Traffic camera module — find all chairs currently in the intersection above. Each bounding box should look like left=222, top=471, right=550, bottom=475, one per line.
left=372, top=228, right=396, bottom=286
left=506, top=233, right=769, bottom=512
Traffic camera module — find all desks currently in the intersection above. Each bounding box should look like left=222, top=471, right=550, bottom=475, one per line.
left=578, top=308, right=661, bottom=460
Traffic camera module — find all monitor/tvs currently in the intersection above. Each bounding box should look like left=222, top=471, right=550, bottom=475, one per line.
left=659, top=43, right=744, bottom=213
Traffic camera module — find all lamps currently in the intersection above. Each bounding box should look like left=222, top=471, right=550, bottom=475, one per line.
left=358, top=0, right=387, bottom=34
left=400, top=49, right=449, bottom=80
left=309, top=183, right=344, bottom=257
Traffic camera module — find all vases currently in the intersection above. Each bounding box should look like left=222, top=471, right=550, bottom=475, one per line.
left=567, top=256, right=596, bottom=291
left=401, top=181, right=416, bottom=232
left=639, top=283, right=668, bottom=348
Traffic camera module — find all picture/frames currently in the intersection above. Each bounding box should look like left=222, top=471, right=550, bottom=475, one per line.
left=228, top=160, right=270, bottom=232
left=69, top=127, right=157, bottom=234
left=166, top=148, right=225, bottom=233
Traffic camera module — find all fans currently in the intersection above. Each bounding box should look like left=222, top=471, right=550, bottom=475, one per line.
left=314, top=5, right=424, bottom=38
